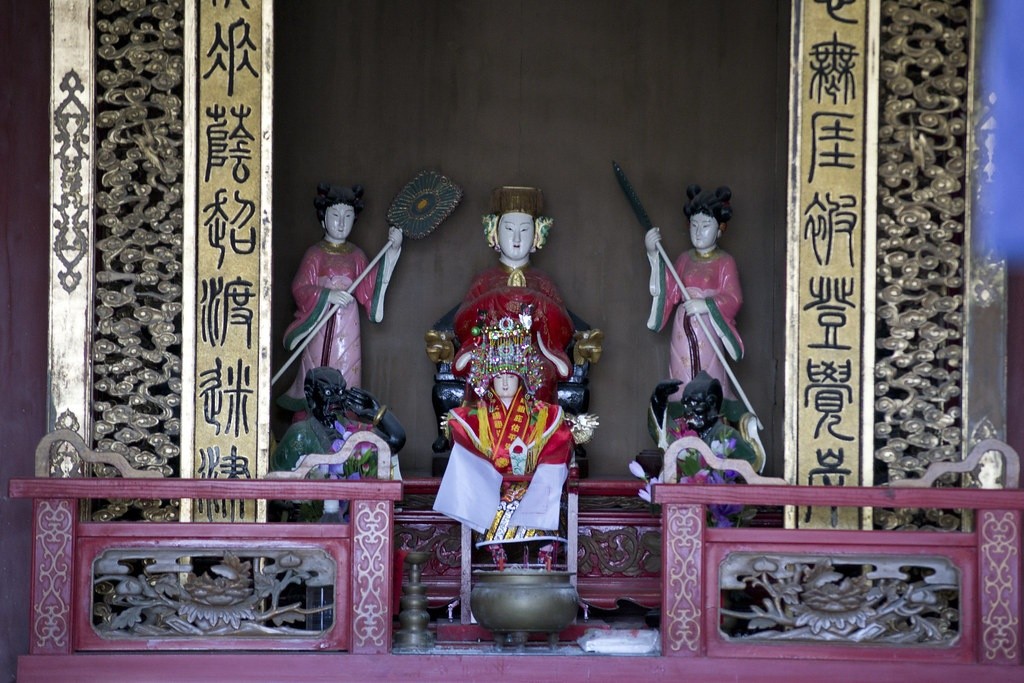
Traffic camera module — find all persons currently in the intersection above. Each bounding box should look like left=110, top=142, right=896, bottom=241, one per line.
left=268, top=367, right=406, bottom=511
left=451, top=184, right=573, bottom=381
left=441, top=308, right=600, bottom=545
left=275, top=182, right=403, bottom=425
left=647, top=367, right=758, bottom=483
left=643, top=184, right=743, bottom=401
